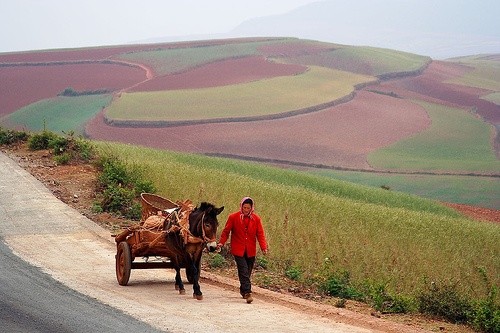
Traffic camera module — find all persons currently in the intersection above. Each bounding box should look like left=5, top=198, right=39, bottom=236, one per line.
left=217, top=197, right=267, bottom=304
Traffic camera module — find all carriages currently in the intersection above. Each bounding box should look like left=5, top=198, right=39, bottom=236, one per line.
left=112, top=202, right=225, bottom=300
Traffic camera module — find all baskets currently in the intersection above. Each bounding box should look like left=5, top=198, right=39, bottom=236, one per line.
left=141, top=192, right=178, bottom=221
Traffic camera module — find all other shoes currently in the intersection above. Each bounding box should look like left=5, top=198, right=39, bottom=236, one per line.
left=243, top=293, right=253, bottom=303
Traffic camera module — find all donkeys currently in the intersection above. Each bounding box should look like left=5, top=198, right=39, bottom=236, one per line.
left=163, top=202, right=225, bottom=300
